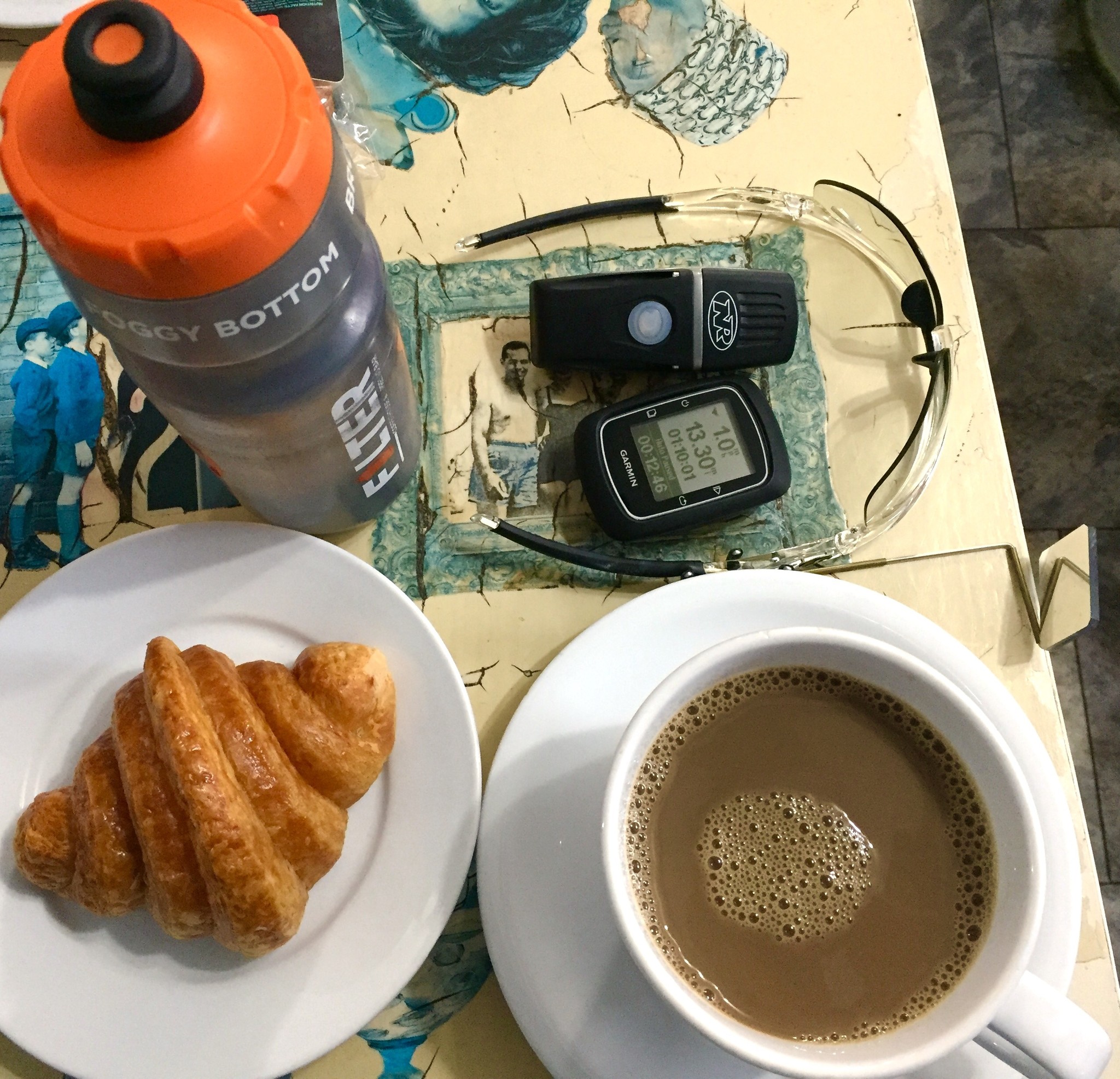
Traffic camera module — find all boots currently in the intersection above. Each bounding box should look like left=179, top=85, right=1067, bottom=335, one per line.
left=76, top=497, right=94, bottom=556
left=57, top=502, right=84, bottom=568
left=4, top=504, right=50, bottom=570
left=29, top=502, right=58, bottom=559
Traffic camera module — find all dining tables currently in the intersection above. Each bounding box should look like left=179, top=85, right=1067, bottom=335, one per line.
left=0, top=0, right=1120, bottom=1079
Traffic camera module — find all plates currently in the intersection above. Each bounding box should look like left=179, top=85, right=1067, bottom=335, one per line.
left=0, top=521, right=489, bottom=1079
left=475, top=567, right=1084, bottom=1079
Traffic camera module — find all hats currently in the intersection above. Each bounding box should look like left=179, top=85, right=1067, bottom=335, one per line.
left=16, top=318, right=49, bottom=350
left=47, top=301, right=82, bottom=337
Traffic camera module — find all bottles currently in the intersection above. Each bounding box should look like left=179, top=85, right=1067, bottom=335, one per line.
left=1, top=2, right=431, bottom=538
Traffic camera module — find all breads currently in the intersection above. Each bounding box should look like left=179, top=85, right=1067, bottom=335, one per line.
left=15, top=637, right=395, bottom=953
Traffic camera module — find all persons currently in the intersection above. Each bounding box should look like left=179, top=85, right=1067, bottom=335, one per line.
left=0, top=299, right=106, bottom=569
left=467, top=338, right=661, bottom=520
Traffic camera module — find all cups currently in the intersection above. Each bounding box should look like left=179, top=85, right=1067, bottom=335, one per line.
left=597, top=630, right=1113, bottom=1079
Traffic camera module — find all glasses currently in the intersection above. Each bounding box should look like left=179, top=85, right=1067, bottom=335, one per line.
left=453, top=178, right=953, bottom=582
